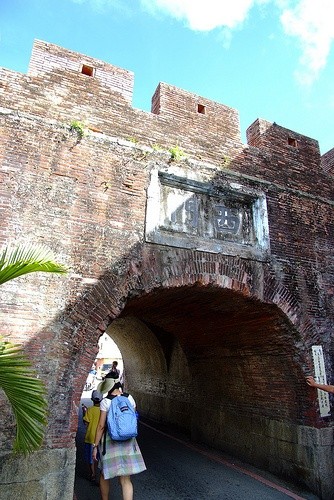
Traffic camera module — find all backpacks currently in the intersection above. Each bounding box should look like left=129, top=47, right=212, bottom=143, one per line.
left=105, top=392, right=138, bottom=441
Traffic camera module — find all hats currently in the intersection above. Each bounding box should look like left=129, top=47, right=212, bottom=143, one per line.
left=92, top=390, right=103, bottom=401
left=97, top=378, right=120, bottom=393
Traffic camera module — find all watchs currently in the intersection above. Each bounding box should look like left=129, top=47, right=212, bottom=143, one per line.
left=92, top=443, right=98, bottom=448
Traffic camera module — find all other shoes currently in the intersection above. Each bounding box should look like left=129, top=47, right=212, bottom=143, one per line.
left=90, top=473, right=96, bottom=481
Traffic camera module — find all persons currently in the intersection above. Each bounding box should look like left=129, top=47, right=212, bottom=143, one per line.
left=92, top=371, right=147, bottom=500
left=82, top=404, right=89, bottom=425
left=82, top=390, right=104, bottom=481
left=109, top=360, right=120, bottom=375
